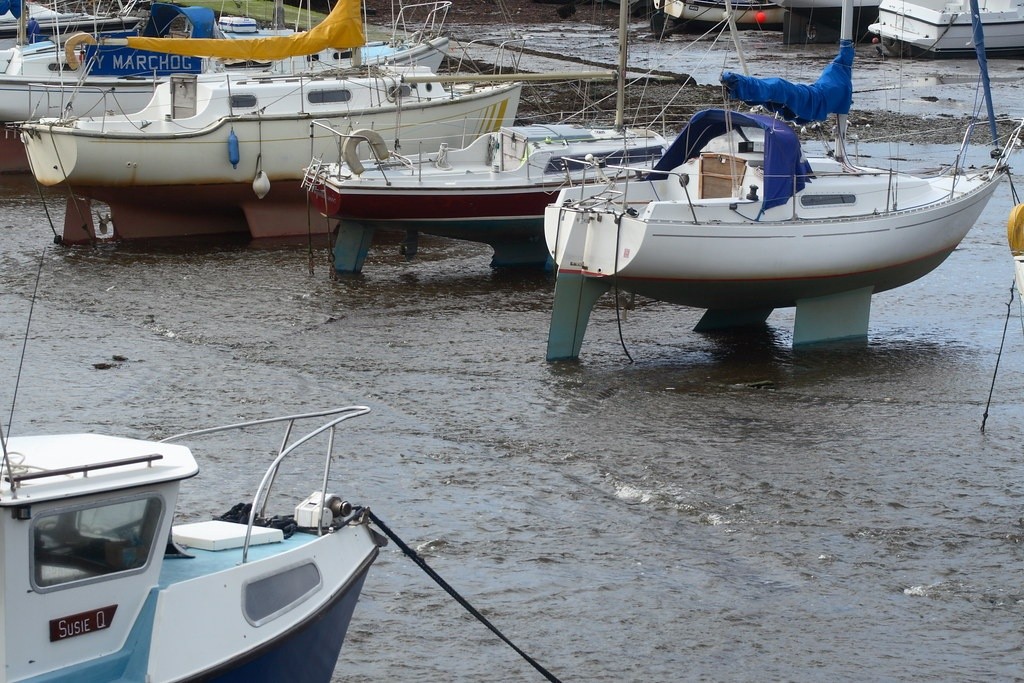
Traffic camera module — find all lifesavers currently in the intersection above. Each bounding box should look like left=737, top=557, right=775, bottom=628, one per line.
left=342, top=129, right=389, bottom=176
left=65, top=32, right=96, bottom=70
left=1008, top=204, right=1024, bottom=255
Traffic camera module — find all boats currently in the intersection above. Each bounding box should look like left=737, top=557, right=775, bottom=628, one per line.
left=0, top=245, right=388, bottom=683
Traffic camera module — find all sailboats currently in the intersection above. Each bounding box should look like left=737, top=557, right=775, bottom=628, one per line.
left=0, top=1, right=1024, bottom=362
